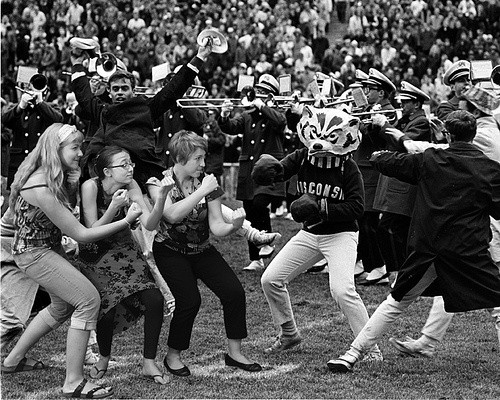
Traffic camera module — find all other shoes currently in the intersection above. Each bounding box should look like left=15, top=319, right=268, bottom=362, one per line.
left=269, top=206, right=294, bottom=221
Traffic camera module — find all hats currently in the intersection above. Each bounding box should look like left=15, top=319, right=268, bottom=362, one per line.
left=462, top=85, right=496, bottom=115
left=69, top=37, right=96, bottom=50
left=307, top=68, right=397, bottom=94
left=444, top=59, right=470, bottom=86
left=394, top=81, right=430, bottom=103
left=256, top=74, right=280, bottom=95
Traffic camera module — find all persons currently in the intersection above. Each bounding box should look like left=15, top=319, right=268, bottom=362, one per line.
left=0, top=0, right=500, bottom=398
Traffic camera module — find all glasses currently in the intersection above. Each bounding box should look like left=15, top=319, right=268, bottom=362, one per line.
left=402, top=100, right=414, bottom=103
left=440, top=129, right=448, bottom=137
left=456, top=78, right=470, bottom=82
left=107, top=163, right=135, bottom=169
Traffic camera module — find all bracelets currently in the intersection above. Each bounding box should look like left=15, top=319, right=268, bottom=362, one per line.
left=399, top=134, right=412, bottom=144
left=124, top=218, right=131, bottom=227
left=248, top=227, right=255, bottom=240
left=251, top=230, right=258, bottom=242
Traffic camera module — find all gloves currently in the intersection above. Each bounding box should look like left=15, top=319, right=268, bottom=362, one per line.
left=252, top=154, right=284, bottom=186
left=291, top=195, right=327, bottom=228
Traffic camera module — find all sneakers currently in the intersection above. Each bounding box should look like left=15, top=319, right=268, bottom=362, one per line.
left=389, top=337, right=421, bottom=357
left=362, top=345, right=383, bottom=362
left=326, top=355, right=356, bottom=373
left=252, top=230, right=282, bottom=248
left=242, top=245, right=275, bottom=271
left=264, top=333, right=302, bottom=354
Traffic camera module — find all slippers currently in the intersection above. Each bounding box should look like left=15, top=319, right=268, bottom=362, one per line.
left=1, top=357, right=48, bottom=373
left=143, top=373, right=170, bottom=385
left=89, top=365, right=107, bottom=380
left=63, top=379, right=114, bottom=399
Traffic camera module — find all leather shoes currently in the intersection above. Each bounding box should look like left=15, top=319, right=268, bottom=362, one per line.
left=164, top=354, right=190, bottom=376
left=308, top=263, right=391, bottom=286
left=225, top=353, right=262, bottom=372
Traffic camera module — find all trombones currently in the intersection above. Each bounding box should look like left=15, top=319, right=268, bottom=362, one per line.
left=1, top=74, right=50, bottom=108
left=131, top=86, right=404, bottom=129
